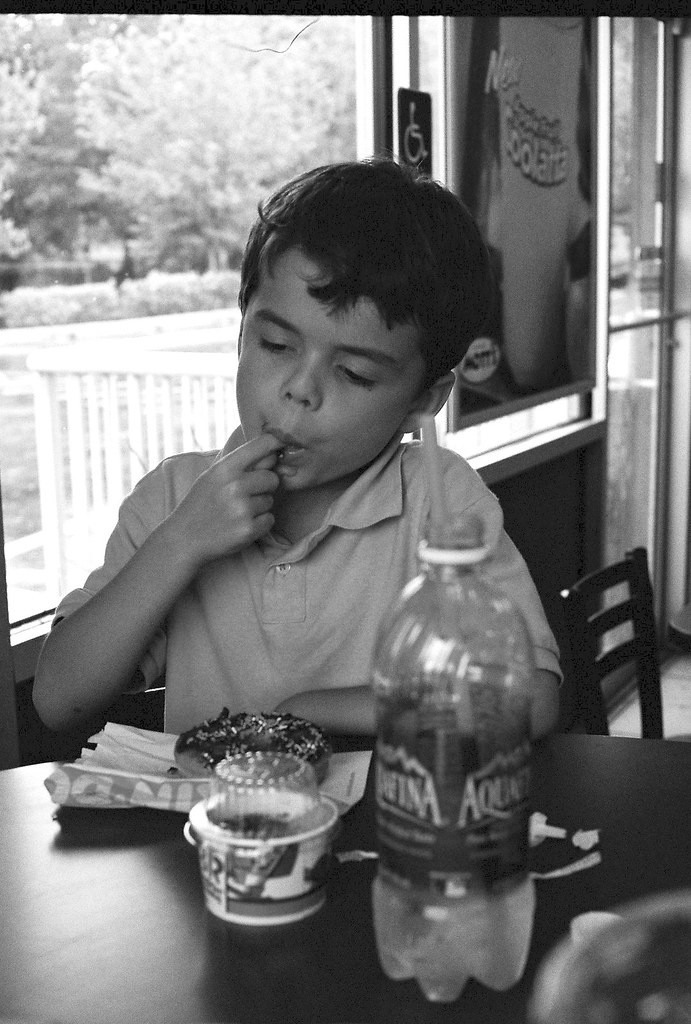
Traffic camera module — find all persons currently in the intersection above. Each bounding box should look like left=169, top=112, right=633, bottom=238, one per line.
left=33, top=161, right=564, bottom=742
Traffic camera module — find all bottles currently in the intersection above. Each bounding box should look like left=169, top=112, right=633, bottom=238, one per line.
left=371, top=509, right=533, bottom=1005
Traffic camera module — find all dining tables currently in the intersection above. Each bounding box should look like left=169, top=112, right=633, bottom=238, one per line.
left=0, top=732, right=691, bottom=1024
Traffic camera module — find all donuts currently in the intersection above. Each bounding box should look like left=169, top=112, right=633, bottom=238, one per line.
left=174, top=707, right=331, bottom=791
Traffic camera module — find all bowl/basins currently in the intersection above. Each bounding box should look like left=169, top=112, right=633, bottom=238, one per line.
left=188, top=793, right=340, bottom=927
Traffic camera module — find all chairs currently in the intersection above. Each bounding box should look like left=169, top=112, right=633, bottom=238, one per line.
left=561, top=545, right=691, bottom=742
